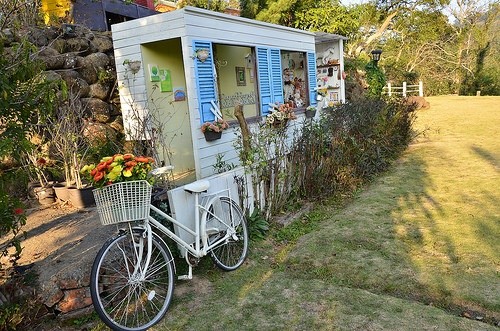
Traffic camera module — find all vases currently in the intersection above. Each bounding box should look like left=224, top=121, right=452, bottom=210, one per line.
left=205, top=130, right=221, bottom=142
left=275, top=118, right=288, bottom=127
left=306, top=111, right=315, bottom=117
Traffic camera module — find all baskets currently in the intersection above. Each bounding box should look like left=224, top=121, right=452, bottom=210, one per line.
left=92, top=180, right=152, bottom=225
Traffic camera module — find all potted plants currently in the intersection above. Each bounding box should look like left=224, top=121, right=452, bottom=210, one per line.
left=0, top=35, right=92, bottom=208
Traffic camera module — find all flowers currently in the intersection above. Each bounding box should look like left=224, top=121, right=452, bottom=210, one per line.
left=306, top=105, right=317, bottom=111
left=267, top=102, right=296, bottom=124
left=202, top=119, right=228, bottom=132
left=81, top=154, right=161, bottom=190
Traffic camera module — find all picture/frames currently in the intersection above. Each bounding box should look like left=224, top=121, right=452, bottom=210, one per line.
left=236, top=67, right=247, bottom=86
